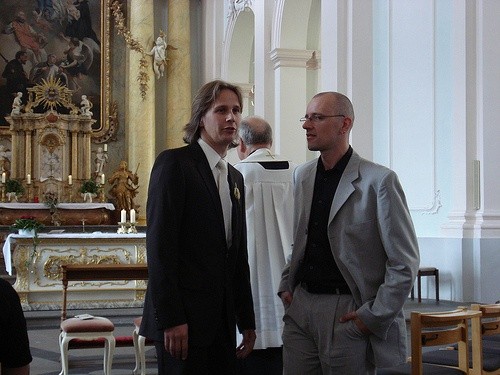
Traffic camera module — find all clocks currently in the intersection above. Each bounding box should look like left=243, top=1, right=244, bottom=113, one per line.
left=3, top=74, right=91, bottom=203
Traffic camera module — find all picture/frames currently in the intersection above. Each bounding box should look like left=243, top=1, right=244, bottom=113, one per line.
left=0, top=0, right=117, bottom=144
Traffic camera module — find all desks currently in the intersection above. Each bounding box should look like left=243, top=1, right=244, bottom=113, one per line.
left=58, top=263, right=147, bottom=362
left=0, top=203, right=115, bottom=244
left=2, top=232, right=147, bottom=311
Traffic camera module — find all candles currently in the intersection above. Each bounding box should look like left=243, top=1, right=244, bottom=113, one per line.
left=1, top=172, right=5, bottom=183
left=68, top=175, right=72, bottom=184
left=121, top=209, right=126, bottom=224
left=100, top=174, right=105, bottom=184
left=104, top=144, right=107, bottom=151
left=27, top=175, right=31, bottom=184
left=129, top=209, right=135, bottom=223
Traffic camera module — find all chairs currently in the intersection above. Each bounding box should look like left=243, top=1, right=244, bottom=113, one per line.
left=377, top=300, right=500, bottom=375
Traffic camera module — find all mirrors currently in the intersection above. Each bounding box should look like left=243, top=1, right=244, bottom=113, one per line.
left=223, top=1, right=255, bottom=137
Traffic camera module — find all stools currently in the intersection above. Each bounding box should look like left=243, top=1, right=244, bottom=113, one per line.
left=411, top=267, right=439, bottom=303
left=132, top=316, right=147, bottom=375
left=58, top=317, right=115, bottom=375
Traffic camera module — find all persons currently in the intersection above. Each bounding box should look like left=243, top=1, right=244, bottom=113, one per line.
left=277, top=91, right=421, bottom=375
left=228, top=116, right=303, bottom=375
left=0, top=277, right=33, bottom=375
left=10, top=92, right=23, bottom=116
left=137, top=80, right=257, bottom=375
left=144, top=34, right=168, bottom=79
left=95, top=147, right=109, bottom=173
left=0, top=144, right=8, bottom=173
left=80, top=95, right=93, bottom=115
left=107, top=160, right=139, bottom=211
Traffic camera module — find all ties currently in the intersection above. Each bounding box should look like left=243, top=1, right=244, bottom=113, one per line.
left=217, top=159, right=232, bottom=246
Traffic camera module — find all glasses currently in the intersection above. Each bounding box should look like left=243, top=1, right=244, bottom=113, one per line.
left=300, top=113, right=346, bottom=124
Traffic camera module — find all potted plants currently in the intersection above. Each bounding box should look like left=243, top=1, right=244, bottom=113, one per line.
left=4, top=178, right=25, bottom=203
left=80, top=178, right=100, bottom=203
left=9, top=216, right=42, bottom=237
